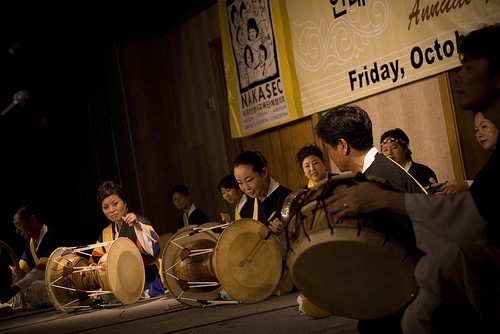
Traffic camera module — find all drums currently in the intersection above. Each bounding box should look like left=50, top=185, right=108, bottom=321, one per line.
left=161, top=218, right=281, bottom=307
left=286, top=173, right=420, bottom=321
left=44, top=237, right=146, bottom=312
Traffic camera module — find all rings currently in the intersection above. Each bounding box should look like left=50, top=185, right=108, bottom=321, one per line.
left=343, top=203, right=347, bottom=208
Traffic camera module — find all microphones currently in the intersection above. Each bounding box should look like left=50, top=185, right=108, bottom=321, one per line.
left=0, top=90, right=30, bottom=118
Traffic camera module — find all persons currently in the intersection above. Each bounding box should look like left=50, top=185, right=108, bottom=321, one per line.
left=314, top=105, right=431, bottom=194
left=325, top=23, right=500, bottom=334
left=218, top=174, right=245, bottom=225
left=11, top=206, right=63, bottom=310
left=233, top=150, right=295, bottom=264
left=436, top=108, right=500, bottom=195
left=89, top=180, right=164, bottom=305
left=378, top=129, right=438, bottom=191
left=169, top=183, right=211, bottom=237
left=295, top=144, right=342, bottom=193
